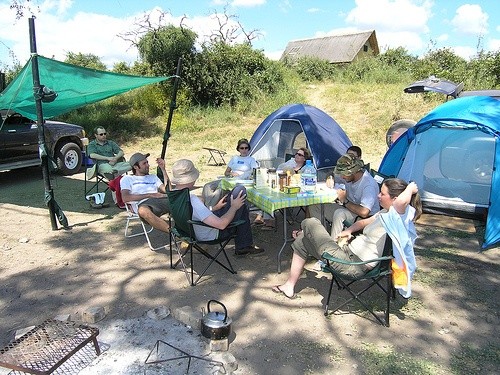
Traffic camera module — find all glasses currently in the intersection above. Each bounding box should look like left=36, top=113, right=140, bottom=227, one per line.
left=96, top=133, right=107, bottom=136
left=295, top=153, right=305, bottom=157
left=239, top=147, right=249, bottom=149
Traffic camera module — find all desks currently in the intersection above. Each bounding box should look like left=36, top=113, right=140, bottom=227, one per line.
left=218, top=176, right=339, bottom=274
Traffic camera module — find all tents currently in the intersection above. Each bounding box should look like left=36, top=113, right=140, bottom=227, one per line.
left=378, top=96, right=500, bottom=250
left=247, top=104, right=353, bottom=181
left=0, top=18, right=182, bottom=230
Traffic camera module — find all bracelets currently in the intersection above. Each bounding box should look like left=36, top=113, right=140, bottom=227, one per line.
left=210, top=206, right=212, bottom=210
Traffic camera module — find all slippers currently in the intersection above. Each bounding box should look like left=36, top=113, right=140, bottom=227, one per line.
left=272, top=285, right=297, bottom=299
left=261, top=224, right=275, bottom=231
left=253, top=219, right=265, bottom=224
left=292, top=230, right=302, bottom=239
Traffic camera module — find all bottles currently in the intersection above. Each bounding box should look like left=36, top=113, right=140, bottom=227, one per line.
left=279, top=172, right=288, bottom=191
left=301, top=160, right=318, bottom=195
left=267, top=168, right=277, bottom=188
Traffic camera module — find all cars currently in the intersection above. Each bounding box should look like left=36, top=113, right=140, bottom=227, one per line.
left=403, top=76, right=500, bottom=102
left=0, top=108, right=90, bottom=176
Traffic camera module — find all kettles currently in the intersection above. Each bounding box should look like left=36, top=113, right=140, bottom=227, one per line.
left=200, top=299, right=232, bottom=340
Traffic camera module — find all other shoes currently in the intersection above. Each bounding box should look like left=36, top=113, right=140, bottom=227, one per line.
left=175, top=236, right=189, bottom=250
left=104, top=173, right=114, bottom=180
left=235, top=246, right=265, bottom=257
left=117, top=203, right=126, bottom=209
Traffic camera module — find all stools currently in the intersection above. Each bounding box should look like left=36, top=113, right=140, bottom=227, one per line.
left=202, top=147, right=227, bottom=167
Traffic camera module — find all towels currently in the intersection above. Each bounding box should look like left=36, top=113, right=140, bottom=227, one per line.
left=379, top=204, right=419, bottom=298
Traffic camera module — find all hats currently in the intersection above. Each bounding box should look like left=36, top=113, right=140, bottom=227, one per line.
left=333, top=153, right=361, bottom=176
left=171, top=159, right=199, bottom=184
left=130, top=153, right=150, bottom=166
left=237, top=139, right=251, bottom=151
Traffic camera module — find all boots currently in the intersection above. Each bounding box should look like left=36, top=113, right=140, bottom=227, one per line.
left=102, top=201, right=110, bottom=207
left=89, top=196, right=103, bottom=209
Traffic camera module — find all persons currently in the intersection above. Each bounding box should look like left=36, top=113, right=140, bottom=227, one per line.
left=120, top=153, right=170, bottom=233
left=272, top=178, right=422, bottom=298
left=255, top=148, right=309, bottom=230
left=306, top=146, right=362, bottom=217
left=87, top=126, right=131, bottom=204
left=171, top=159, right=264, bottom=256
left=309, top=153, right=380, bottom=270
left=203, top=138, right=255, bottom=207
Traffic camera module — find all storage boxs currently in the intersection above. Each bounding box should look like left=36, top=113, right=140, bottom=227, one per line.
left=283, top=185, right=300, bottom=194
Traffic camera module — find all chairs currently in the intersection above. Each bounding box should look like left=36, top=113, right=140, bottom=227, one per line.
left=322, top=236, right=395, bottom=328
left=119, top=174, right=183, bottom=250
left=84, top=146, right=129, bottom=202
left=165, top=181, right=249, bottom=286
